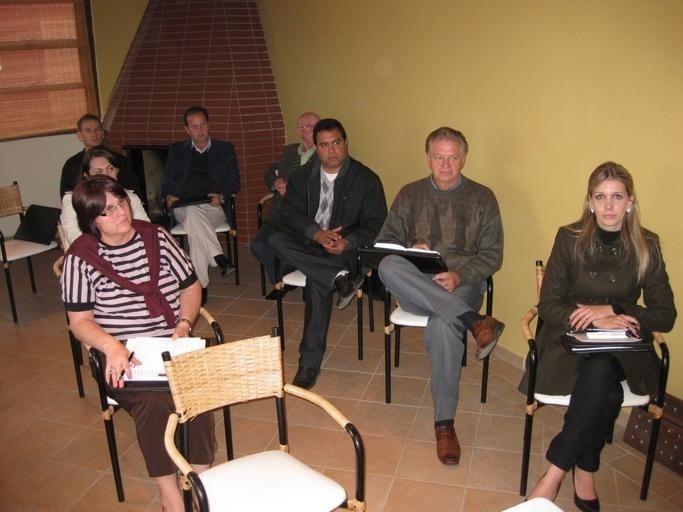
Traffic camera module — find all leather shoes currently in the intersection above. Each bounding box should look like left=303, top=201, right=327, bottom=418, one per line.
left=220, top=262, right=235, bottom=276
left=291, top=366, right=319, bottom=394
left=433, top=424, right=462, bottom=467
left=199, top=287, right=210, bottom=307
left=266, top=288, right=286, bottom=301
left=336, top=274, right=367, bottom=309
left=471, top=313, right=506, bottom=360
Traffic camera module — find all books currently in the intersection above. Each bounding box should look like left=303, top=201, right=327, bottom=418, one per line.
left=119, top=336, right=207, bottom=385
left=559, top=324, right=650, bottom=353
left=357, top=242, right=449, bottom=273
left=173, top=197, right=211, bottom=209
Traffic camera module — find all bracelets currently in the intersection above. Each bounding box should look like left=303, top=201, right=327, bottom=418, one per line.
left=172, top=318, right=193, bottom=331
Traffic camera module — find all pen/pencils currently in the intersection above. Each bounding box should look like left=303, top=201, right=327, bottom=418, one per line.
left=628, top=320, right=641, bottom=326
left=117, top=351, right=135, bottom=378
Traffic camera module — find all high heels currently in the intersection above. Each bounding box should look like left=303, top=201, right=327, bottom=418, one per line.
left=524, top=473, right=562, bottom=505
left=572, top=464, right=600, bottom=512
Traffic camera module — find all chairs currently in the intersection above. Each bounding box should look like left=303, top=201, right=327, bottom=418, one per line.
left=84, top=299, right=235, bottom=502
left=256, top=191, right=276, bottom=294
left=160, top=146, right=241, bottom=286
left=385, top=274, right=494, bottom=404
left=161, top=333, right=366, bottom=510
left=52, top=256, right=87, bottom=398
left=518, top=259, right=672, bottom=501
left=274, top=269, right=374, bottom=360
left=0, top=181, right=61, bottom=321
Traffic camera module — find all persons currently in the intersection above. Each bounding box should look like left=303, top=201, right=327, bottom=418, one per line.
left=267, top=117, right=387, bottom=393
left=356, top=124, right=505, bottom=465
left=59, top=173, right=235, bottom=512
left=58, top=147, right=152, bottom=255
left=249, top=111, right=322, bottom=301
left=161, top=106, right=241, bottom=308
left=524, top=160, right=676, bottom=512
left=58, top=113, right=140, bottom=205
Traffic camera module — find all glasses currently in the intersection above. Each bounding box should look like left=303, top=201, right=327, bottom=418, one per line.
left=98, top=197, right=130, bottom=217
left=297, top=124, right=315, bottom=130
